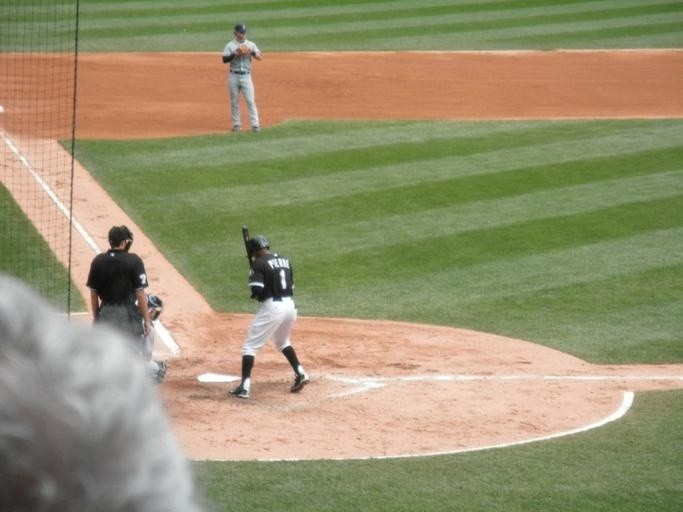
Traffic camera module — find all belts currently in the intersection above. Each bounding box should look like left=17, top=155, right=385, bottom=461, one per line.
left=230, top=69, right=250, bottom=75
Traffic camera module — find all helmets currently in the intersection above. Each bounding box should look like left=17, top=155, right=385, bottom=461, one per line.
left=142, top=295, right=163, bottom=321
left=246, top=235, right=269, bottom=254
left=234, top=24, right=247, bottom=33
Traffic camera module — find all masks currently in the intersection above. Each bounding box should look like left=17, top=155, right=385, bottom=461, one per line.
left=124, top=238, right=133, bottom=251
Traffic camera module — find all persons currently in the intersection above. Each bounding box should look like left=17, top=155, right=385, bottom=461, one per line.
left=228, top=235, right=311, bottom=399
left=85, top=223, right=167, bottom=384
left=222, top=24, right=264, bottom=133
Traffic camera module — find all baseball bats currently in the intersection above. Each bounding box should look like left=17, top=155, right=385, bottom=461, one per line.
left=242, top=226, right=253, bottom=275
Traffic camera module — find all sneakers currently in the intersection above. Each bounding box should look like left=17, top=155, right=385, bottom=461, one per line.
left=228, top=386, right=251, bottom=400
left=290, top=373, right=311, bottom=393
left=232, top=125, right=260, bottom=132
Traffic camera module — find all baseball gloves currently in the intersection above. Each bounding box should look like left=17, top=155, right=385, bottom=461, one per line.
left=236, top=44, right=251, bottom=55
